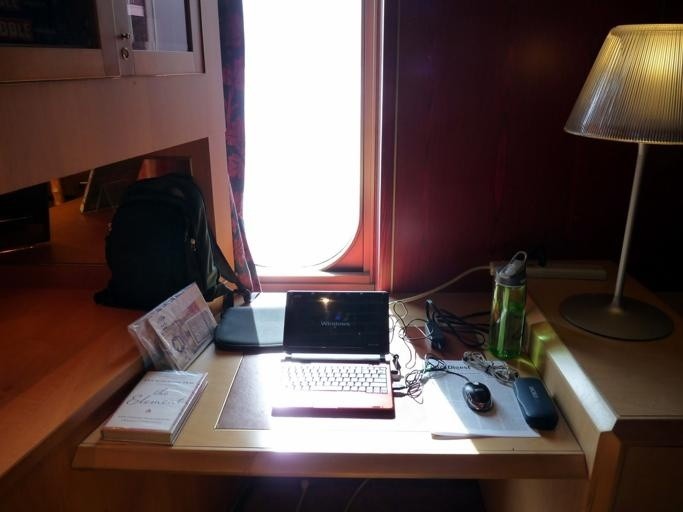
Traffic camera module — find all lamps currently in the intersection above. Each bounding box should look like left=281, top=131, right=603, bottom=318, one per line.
left=559, top=23, right=683, bottom=342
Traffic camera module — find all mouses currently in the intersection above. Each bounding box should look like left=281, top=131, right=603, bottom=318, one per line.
left=463, top=380, right=493, bottom=413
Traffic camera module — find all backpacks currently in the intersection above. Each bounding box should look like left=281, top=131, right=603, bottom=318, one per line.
left=94, top=171, right=220, bottom=310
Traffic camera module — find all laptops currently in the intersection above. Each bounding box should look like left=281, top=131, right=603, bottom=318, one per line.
left=272, top=290, right=395, bottom=414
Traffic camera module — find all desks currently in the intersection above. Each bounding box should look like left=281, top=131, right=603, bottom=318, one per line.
left=70, top=294, right=588, bottom=482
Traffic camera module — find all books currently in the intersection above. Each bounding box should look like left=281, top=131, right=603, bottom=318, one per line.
left=97, top=367, right=211, bottom=446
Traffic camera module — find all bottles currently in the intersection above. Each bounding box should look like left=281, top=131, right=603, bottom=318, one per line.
left=488, top=250, right=528, bottom=361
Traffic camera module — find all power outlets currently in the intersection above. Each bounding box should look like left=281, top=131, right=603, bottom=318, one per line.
left=488, top=260, right=564, bottom=281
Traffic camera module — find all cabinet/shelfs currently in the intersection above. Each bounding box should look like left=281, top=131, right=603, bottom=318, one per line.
left=479, top=258, right=683, bottom=512
left=0, top=0, right=236, bottom=498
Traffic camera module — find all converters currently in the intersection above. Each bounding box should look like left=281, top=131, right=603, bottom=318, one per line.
left=426, top=320, right=446, bottom=351
